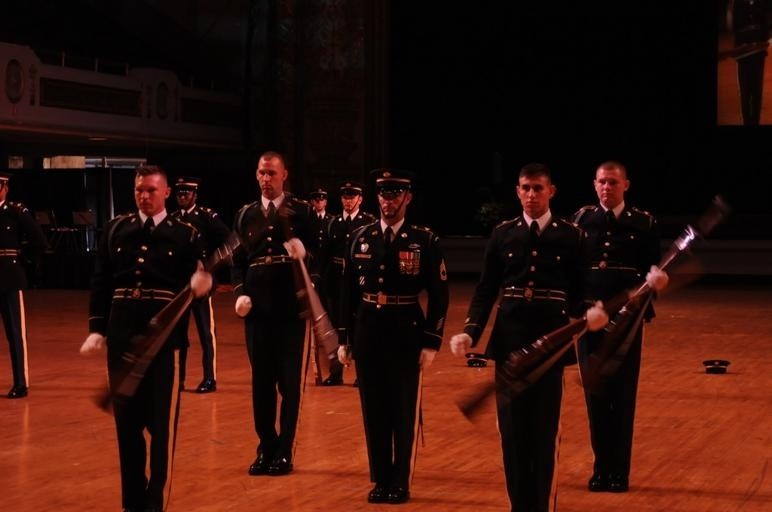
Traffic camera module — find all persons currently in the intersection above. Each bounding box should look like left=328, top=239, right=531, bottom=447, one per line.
left=340, top=166, right=449, bottom=504
left=79, top=165, right=215, bottom=512
left=229, top=152, right=324, bottom=477
left=307, top=184, right=336, bottom=387
left=167, top=176, right=231, bottom=393
left=449, top=160, right=584, bottom=512
left=0, top=173, right=48, bottom=399
left=322, top=178, right=377, bottom=388
left=568, top=161, right=669, bottom=493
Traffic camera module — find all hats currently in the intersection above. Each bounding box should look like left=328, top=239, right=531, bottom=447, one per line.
left=702, top=358, right=731, bottom=376
left=173, top=176, right=203, bottom=192
left=367, top=166, right=413, bottom=195
left=337, top=180, right=367, bottom=196
left=308, top=184, right=330, bottom=200
left=465, top=352, right=490, bottom=368
left=1, top=171, right=13, bottom=186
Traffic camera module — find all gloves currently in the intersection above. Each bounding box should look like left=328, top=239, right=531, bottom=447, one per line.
left=336, top=343, right=354, bottom=370
left=585, top=298, right=610, bottom=332
left=78, top=331, right=108, bottom=358
left=448, top=332, right=474, bottom=358
left=417, top=346, right=439, bottom=372
left=234, top=294, right=253, bottom=319
left=188, top=269, right=214, bottom=300
left=282, top=237, right=307, bottom=261
left=644, top=264, right=670, bottom=293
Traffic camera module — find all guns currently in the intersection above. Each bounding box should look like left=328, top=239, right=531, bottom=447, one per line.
left=92, top=234, right=242, bottom=416
left=453, top=303, right=626, bottom=425
left=290, top=220, right=338, bottom=359
left=600, top=227, right=694, bottom=334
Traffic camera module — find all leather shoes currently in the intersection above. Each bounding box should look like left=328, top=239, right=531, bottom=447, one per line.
left=387, top=485, right=409, bottom=504
left=587, top=473, right=610, bottom=492
left=7, top=384, right=29, bottom=399
left=322, top=375, right=345, bottom=387
left=368, top=483, right=392, bottom=504
left=194, top=378, right=217, bottom=395
left=608, top=474, right=629, bottom=493
left=248, top=448, right=274, bottom=476
left=266, top=452, right=293, bottom=476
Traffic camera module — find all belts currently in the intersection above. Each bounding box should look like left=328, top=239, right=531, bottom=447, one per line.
left=329, top=256, right=344, bottom=265
left=586, top=259, right=639, bottom=275
left=112, top=287, right=180, bottom=304
left=248, top=254, right=293, bottom=268
left=0, top=247, right=22, bottom=258
left=361, top=291, right=419, bottom=307
left=502, top=286, right=568, bottom=303
